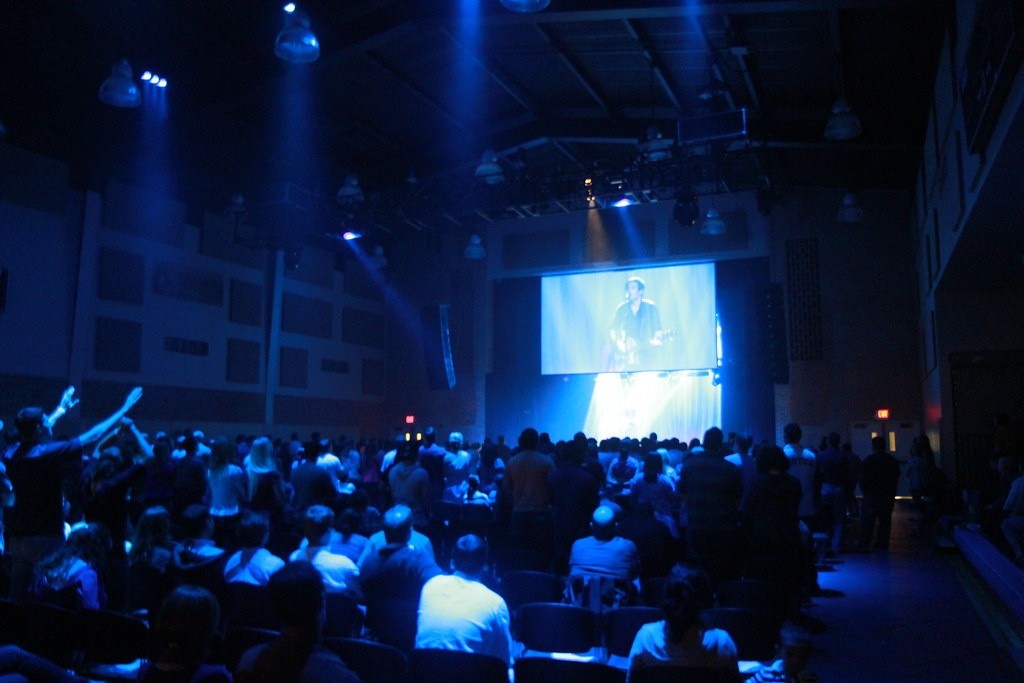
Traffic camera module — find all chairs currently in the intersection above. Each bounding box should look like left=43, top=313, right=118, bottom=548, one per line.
left=0, top=500, right=750, bottom=683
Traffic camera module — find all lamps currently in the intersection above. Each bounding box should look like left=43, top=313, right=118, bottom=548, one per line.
left=823, top=94, right=864, bottom=141
left=97, top=56, right=143, bottom=109
left=700, top=194, right=727, bottom=236
left=273, top=0, right=321, bottom=64
left=835, top=192, right=876, bottom=222
left=463, top=231, right=487, bottom=260
left=876, top=407, right=892, bottom=439
left=133, top=54, right=176, bottom=89
left=474, top=147, right=505, bottom=185
left=336, top=171, right=362, bottom=198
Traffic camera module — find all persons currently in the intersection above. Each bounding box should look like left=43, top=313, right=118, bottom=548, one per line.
left=87, top=585, right=232, bottom=683
left=359, top=505, right=442, bottom=649
left=861, top=437, right=902, bottom=550
left=28, top=527, right=102, bottom=618
left=0, top=645, right=90, bottom=683
left=741, top=628, right=819, bottom=683
left=609, top=276, right=664, bottom=371
left=0, top=386, right=861, bottom=603
left=411, top=534, right=513, bottom=667
left=625, top=578, right=741, bottom=683
left=904, top=437, right=937, bottom=537
left=235, top=558, right=362, bottom=683
left=1000, top=461, right=1024, bottom=560
